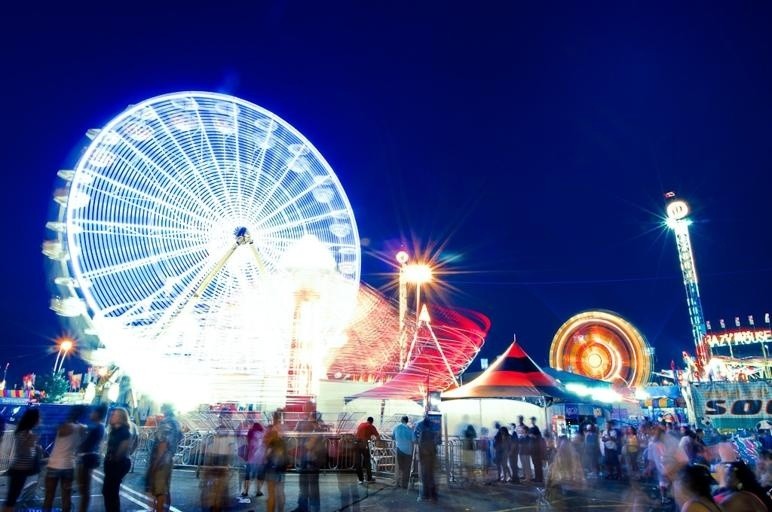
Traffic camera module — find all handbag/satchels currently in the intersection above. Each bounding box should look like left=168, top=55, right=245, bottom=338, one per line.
left=76, top=451, right=100, bottom=475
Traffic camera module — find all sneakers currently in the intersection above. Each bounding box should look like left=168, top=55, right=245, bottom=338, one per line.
left=496, top=476, right=543, bottom=484
left=241, top=490, right=248, bottom=496
left=417, top=497, right=439, bottom=506
left=359, top=476, right=376, bottom=484
left=255, top=490, right=264, bottom=497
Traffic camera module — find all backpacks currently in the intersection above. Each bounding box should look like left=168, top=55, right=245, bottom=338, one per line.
left=420, top=431, right=437, bottom=457
